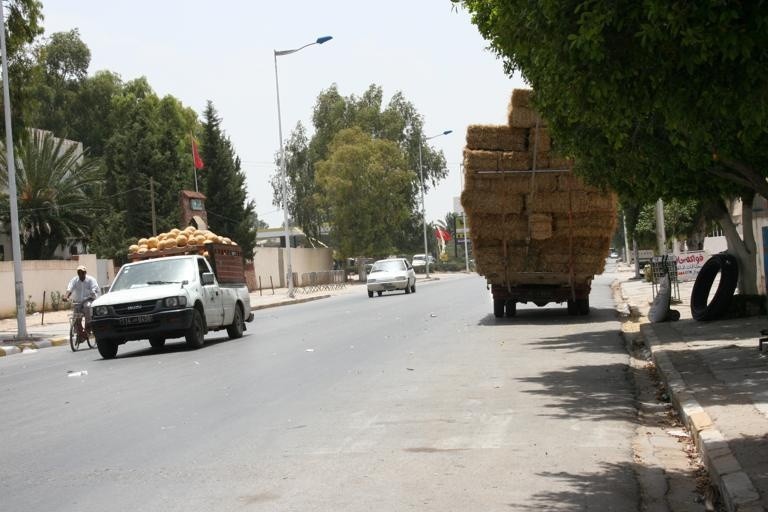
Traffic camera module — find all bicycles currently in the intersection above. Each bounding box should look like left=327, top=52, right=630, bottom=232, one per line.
left=61, top=299, right=97, bottom=352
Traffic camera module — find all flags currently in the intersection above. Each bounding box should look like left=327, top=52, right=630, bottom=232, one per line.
left=437, top=227, right=451, bottom=241
left=193, top=142, right=204, bottom=168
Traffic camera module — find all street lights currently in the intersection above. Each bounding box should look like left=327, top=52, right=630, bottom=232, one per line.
left=272, top=32, right=334, bottom=296
left=416, top=128, right=453, bottom=280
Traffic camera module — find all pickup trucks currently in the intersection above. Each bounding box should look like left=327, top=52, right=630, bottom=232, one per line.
left=84, top=255, right=253, bottom=365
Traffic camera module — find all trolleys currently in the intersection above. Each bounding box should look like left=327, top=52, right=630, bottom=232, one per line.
left=660, top=253, right=680, bottom=324
left=647, top=258, right=681, bottom=305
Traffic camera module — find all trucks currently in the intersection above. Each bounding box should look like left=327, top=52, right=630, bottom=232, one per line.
left=486, top=281, right=592, bottom=318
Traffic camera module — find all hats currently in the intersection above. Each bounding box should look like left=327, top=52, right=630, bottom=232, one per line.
left=76, top=265, right=86, bottom=272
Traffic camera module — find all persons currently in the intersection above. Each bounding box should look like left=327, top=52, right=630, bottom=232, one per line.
left=63, top=265, right=99, bottom=343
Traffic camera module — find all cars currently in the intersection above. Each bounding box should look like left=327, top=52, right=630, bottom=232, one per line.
left=365, top=257, right=417, bottom=297
left=611, top=252, right=619, bottom=258
left=347, top=253, right=436, bottom=274
